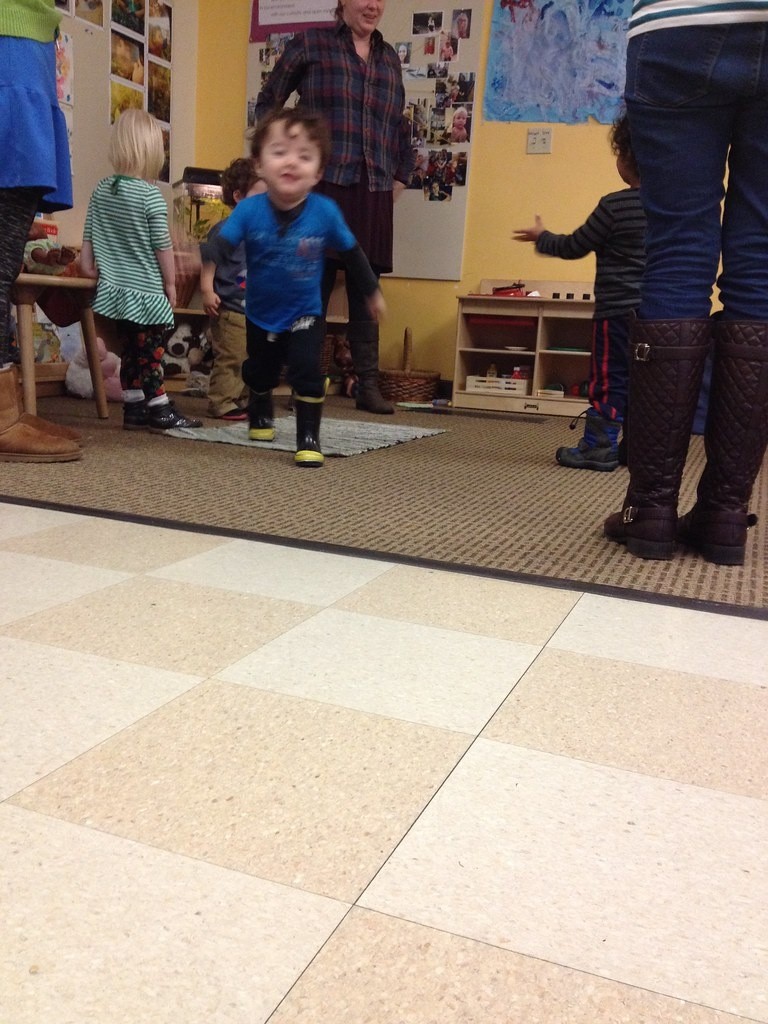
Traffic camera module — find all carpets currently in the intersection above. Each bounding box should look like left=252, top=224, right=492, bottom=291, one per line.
left=1, top=394, right=768, bottom=622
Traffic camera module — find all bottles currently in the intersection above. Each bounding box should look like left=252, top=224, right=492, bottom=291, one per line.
left=487, top=362, right=532, bottom=388
left=430, top=399, right=448, bottom=405
left=571, top=380, right=589, bottom=396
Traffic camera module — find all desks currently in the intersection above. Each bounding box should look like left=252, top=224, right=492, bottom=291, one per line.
left=163, top=308, right=209, bottom=392
left=10, top=272, right=108, bottom=419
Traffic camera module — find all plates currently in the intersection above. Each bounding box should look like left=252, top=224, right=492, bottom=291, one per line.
left=505, top=346, right=528, bottom=351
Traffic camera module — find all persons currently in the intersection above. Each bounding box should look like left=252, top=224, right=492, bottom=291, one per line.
left=198, top=108, right=385, bottom=468
left=511, top=109, right=645, bottom=469
left=251, top=0, right=415, bottom=416
left=110, top=0, right=169, bottom=123
left=605, top=1, right=768, bottom=563
left=260, top=13, right=474, bottom=203
left=82, top=109, right=201, bottom=432
left=1, top=0, right=89, bottom=463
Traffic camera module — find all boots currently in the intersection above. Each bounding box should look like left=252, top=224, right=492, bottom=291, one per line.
left=676, top=318, right=768, bottom=565
left=293, top=377, right=332, bottom=467
left=0, top=362, right=84, bottom=463
left=605, top=319, right=712, bottom=560
left=247, top=387, right=275, bottom=440
left=556, top=410, right=627, bottom=471
left=346, top=320, right=394, bottom=414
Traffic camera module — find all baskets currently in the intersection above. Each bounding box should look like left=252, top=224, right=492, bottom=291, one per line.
left=378, top=326, right=442, bottom=403
left=321, top=334, right=334, bottom=376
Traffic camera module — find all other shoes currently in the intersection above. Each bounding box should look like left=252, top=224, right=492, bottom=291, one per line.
left=219, top=408, right=247, bottom=420
left=122, top=398, right=151, bottom=429
left=147, top=400, right=202, bottom=428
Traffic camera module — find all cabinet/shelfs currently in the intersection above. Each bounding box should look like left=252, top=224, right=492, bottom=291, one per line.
left=455, top=279, right=596, bottom=418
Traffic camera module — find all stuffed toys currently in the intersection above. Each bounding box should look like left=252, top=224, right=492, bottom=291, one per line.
left=19, top=221, right=210, bottom=403
